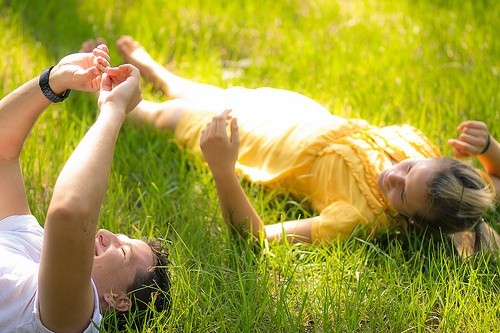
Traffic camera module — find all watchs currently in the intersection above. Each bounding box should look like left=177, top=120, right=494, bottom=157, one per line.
left=38, top=64, right=71, bottom=102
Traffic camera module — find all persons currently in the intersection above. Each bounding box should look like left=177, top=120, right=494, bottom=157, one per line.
left=0, top=44, right=173, bottom=333
left=78, top=33, right=500, bottom=251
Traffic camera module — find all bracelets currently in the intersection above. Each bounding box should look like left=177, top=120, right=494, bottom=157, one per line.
left=480, top=133, right=491, bottom=153
